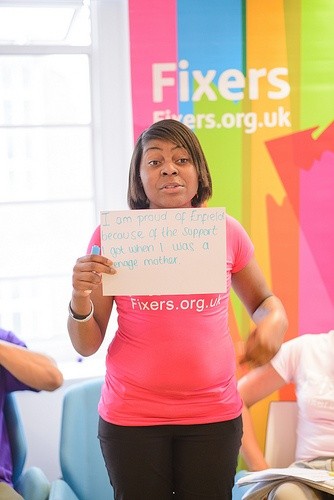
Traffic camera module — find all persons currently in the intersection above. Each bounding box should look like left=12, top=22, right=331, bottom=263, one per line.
left=0, top=326, right=64, bottom=500
left=236, top=329, right=334, bottom=500
left=67, top=119, right=288, bottom=499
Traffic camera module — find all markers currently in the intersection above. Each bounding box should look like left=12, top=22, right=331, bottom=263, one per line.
left=84, top=245, right=100, bottom=294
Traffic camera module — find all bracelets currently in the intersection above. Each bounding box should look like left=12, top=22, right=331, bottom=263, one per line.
left=68, top=297, right=95, bottom=323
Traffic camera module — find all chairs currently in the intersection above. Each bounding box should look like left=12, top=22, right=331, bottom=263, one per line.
left=2, top=392, right=48, bottom=499
left=263, top=399, right=299, bottom=470
left=48, top=381, right=114, bottom=500
